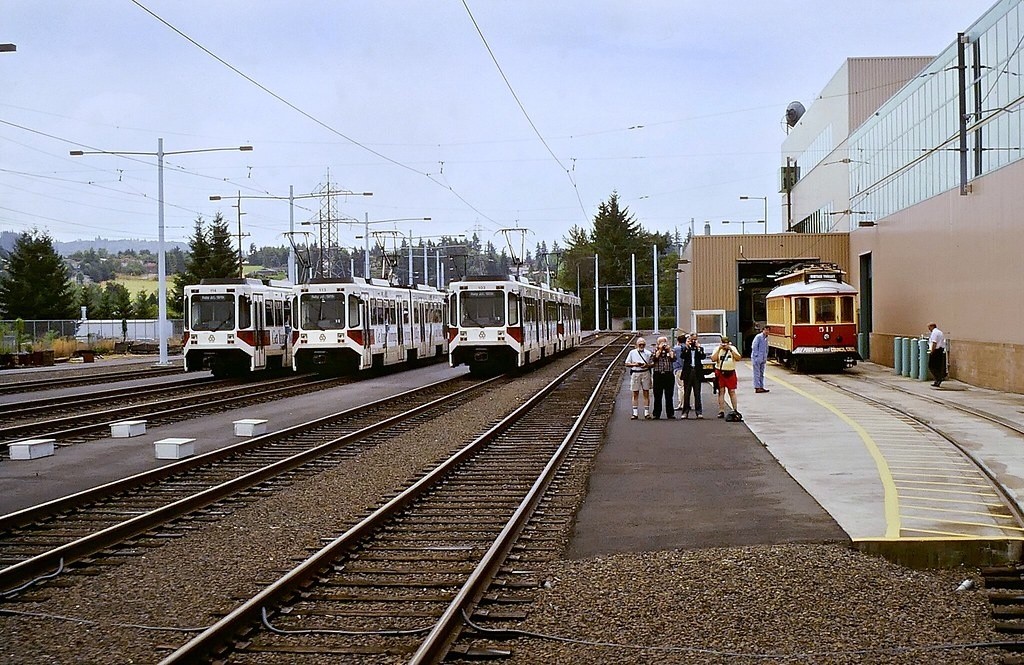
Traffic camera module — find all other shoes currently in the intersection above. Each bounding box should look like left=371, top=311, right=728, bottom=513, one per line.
left=756, top=388, right=769, bottom=393
left=652, top=416, right=660, bottom=420
left=696, top=414, right=703, bottom=418
left=644, top=413, right=651, bottom=419
left=681, top=412, right=688, bottom=418
left=667, top=415, right=676, bottom=419
left=631, top=414, right=638, bottom=420
left=718, top=412, right=725, bottom=418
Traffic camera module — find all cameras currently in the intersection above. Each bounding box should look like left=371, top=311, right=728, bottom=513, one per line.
left=722, top=344, right=727, bottom=350
left=662, top=344, right=667, bottom=347
left=638, top=363, right=644, bottom=365
left=689, top=341, right=696, bottom=345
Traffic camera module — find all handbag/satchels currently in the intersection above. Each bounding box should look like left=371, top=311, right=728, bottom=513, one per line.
left=720, top=370, right=734, bottom=378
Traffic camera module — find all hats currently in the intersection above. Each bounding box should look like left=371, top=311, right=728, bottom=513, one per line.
left=721, top=336, right=731, bottom=343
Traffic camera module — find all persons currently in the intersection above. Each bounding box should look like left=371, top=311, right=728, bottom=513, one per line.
left=927, top=322, right=945, bottom=387
left=625, top=332, right=741, bottom=420
left=751, top=325, right=770, bottom=393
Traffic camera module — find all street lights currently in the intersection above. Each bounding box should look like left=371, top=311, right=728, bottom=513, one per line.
left=69, top=137, right=253, bottom=367
left=721, top=220, right=765, bottom=234
left=740, top=196, right=767, bottom=235
left=209, top=185, right=373, bottom=282
left=356, top=230, right=465, bottom=288
left=301, top=213, right=432, bottom=280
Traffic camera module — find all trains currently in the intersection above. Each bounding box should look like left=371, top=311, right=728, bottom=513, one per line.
left=287, top=275, right=466, bottom=375
left=443, top=273, right=582, bottom=378
left=764, top=260, right=860, bottom=376
left=179, top=276, right=309, bottom=384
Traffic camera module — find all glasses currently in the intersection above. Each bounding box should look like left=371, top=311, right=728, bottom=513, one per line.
left=638, top=344, right=645, bottom=346
